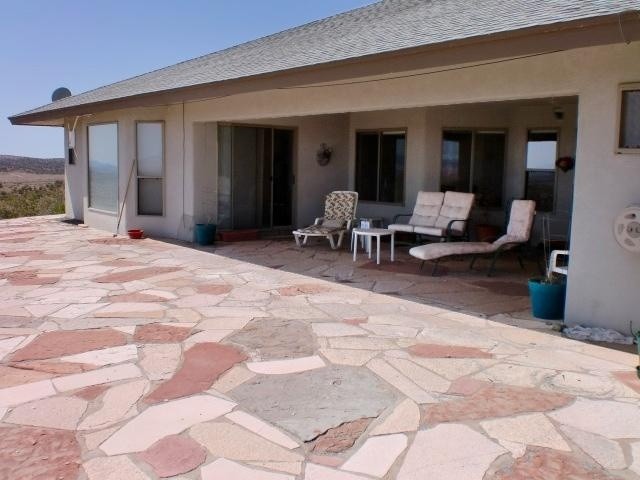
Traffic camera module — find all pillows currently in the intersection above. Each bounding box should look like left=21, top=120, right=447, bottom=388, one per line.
left=321, top=219, right=345, bottom=228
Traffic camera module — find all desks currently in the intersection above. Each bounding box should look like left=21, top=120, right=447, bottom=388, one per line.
left=350, top=227, right=395, bottom=265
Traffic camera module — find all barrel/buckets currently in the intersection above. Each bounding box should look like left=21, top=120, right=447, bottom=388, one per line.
left=528, top=276, right=566, bottom=319
left=195, top=223, right=217, bottom=246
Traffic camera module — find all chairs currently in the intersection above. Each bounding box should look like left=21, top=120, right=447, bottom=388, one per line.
left=408, top=198, right=536, bottom=276
left=546, top=249, right=570, bottom=280
left=292, top=191, right=358, bottom=250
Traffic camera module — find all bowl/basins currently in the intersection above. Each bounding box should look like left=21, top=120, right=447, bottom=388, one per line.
left=127, top=229, right=144, bottom=239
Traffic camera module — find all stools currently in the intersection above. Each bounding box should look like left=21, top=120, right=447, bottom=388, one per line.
left=349, top=215, right=385, bottom=252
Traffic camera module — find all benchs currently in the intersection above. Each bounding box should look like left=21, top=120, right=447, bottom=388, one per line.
left=387, top=191, right=475, bottom=250
